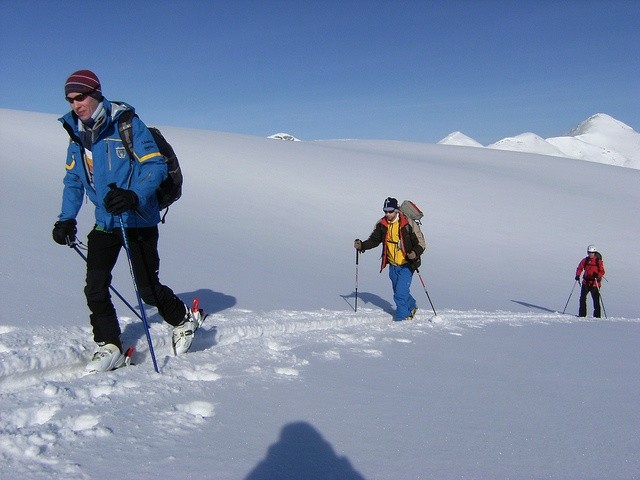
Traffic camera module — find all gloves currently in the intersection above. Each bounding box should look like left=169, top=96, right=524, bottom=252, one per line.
left=355, top=240, right=362, bottom=252
left=52, top=219, right=77, bottom=245
left=104, top=188, right=140, bottom=217
left=575, top=276, right=579, bottom=281
left=407, top=250, right=417, bottom=262
left=593, top=272, right=597, bottom=280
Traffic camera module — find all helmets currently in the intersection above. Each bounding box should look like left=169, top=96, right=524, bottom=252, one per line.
left=587, top=245, right=596, bottom=253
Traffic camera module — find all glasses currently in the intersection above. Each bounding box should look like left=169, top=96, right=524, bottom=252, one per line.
left=385, top=209, right=395, bottom=214
left=65, top=85, right=100, bottom=104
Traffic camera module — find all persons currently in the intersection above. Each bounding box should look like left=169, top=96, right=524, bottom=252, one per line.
left=354, top=197, right=426, bottom=321
left=575, top=245, right=605, bottom=318
left=52, top=70, right=202, bottom=373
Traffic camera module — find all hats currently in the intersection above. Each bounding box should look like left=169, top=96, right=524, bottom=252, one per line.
left=65, top=70, right=101, bottom=100
left=383, top=197, right=398, bottom=212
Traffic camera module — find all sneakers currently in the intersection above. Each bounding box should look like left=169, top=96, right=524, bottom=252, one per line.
left=172, top=307, right=201, bottom=356
left=411, top=307, right=416, bottom=316
left=87, top=343, right=127, bottom=371
left=403, top=316, right=413, bottom=320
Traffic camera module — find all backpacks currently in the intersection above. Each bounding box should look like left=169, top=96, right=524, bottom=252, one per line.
left=399, top=200, right=423, bottom=232
left=585, top=252, right=602, bottom=270
left=118, top=111, right=183, bottom=225
